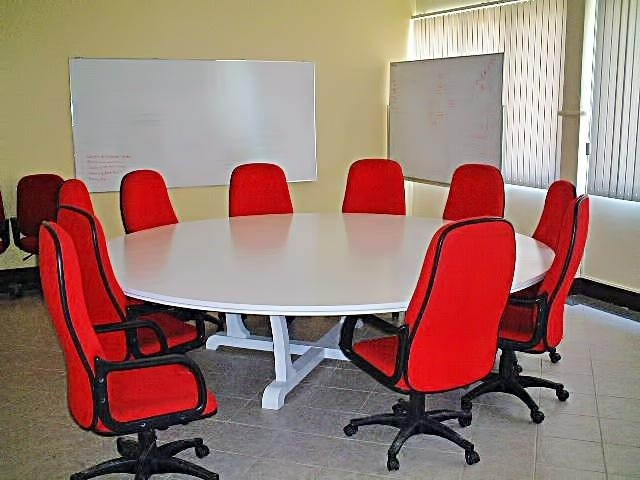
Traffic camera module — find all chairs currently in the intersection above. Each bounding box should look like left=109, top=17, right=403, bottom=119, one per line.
left=0, top=191, right=20, bottom=299
left=39, top=169, right=226, bottom=480
left=10, top=171, right=66, bottom=302
left=338, top=155, right=591, bottom=471
left=228, top=163, right=295, bottom=218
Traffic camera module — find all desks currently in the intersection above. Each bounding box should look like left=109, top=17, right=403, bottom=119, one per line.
left=104, top=211, right=557, bottom=409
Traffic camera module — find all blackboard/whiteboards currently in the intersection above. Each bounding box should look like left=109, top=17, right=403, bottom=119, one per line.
left=67, top=56, right=318, bottom=194
left=388, top=53, right=504, bottom=185
